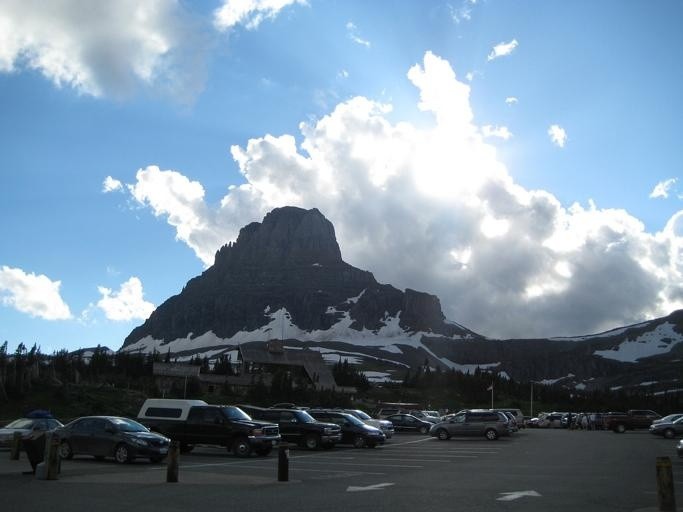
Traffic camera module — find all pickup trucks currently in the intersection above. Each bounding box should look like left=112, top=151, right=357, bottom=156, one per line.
left=605, top=409, right=663, bottom=433
left=135, top=398, right=395, bottom=459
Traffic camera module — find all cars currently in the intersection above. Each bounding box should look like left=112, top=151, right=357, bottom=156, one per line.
left=373, top=408, right=626, bottom=441
left=649, top=413, right=683, bottom=460
left=0, top=415, right=66, bottom=451
left=53, top=415, right=172, bottom=466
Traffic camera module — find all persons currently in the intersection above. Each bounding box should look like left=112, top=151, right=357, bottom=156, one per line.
left=563, top=411, right=609, bottom=431
